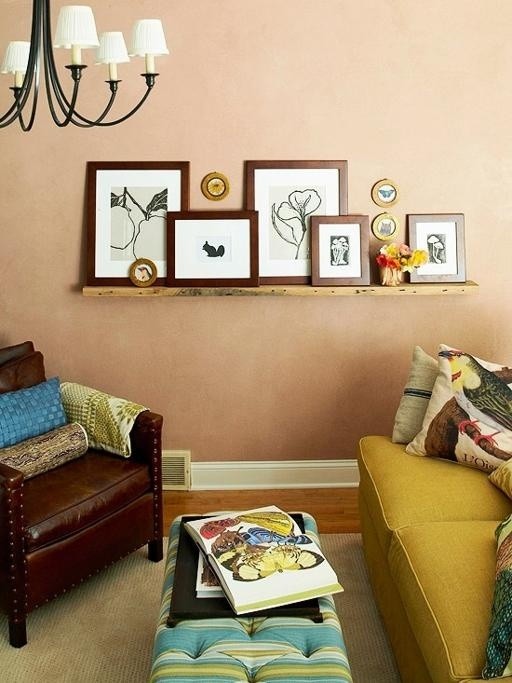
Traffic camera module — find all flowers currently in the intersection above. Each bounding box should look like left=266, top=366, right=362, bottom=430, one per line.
left=376, top=242, right=431, bottom=273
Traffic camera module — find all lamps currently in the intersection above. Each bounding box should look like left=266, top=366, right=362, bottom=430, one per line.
left=0, top=0, right=172, bottom=132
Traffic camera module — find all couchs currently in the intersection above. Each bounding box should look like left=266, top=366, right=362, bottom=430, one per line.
left=1, top=341, right=167, bottom=647
left=358, top=435, right=512, bottom=682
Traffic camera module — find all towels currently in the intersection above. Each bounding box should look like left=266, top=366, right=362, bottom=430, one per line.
left=57, top=380, right=150, bottom=460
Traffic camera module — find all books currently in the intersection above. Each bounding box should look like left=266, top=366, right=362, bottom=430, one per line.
left=183, top=505, right=344, bottom=615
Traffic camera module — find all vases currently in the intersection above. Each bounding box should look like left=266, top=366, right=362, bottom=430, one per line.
left=380, top=266, right=403, bottom=286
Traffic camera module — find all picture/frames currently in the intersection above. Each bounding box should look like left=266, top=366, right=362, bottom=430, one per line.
left=166, top=211, right=260, bottom=287
left=372, top=178, right=400, bottom=207
left=85, top=160, right=189, bottom=286
left=131, top=258, right=157, bottom=287
left=201, top=172, right=230, bottom=200
left=406, top=214, right=467, bottom=284
left=310, top=215, right=370, bottom=286
left=243, top=159, right=348, bottom=285
left=372, top=213, right=399, bottom=241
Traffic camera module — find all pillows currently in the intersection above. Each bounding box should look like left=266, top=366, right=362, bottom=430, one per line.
left=0, top=421, right=89, bottom=477
left=481, top=511, right=512, bottom=682
left=405, top=343, right=512, bottom=468
left=392, top=345, right=440, bottom=444
left=0, top=377, right=68, bottom=448
left=485, top=455, right=512, bottom=502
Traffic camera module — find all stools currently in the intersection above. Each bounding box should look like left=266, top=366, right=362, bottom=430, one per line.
left=148, top=508, right=349, bottom=683
